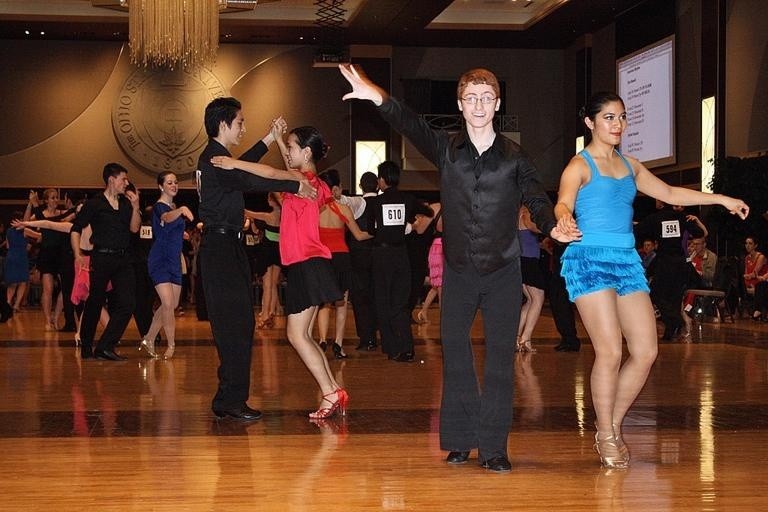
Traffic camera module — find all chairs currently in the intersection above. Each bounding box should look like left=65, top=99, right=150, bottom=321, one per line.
left=684, top=255, right=742, bottom=326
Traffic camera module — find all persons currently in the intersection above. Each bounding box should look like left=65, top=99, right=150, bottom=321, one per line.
left=194, top=97, right=318, bottom=422
left=208, top=116, right=350, bottom=419
left=553, top=90, right=751, bottom=470
left=336, top=63, right=584, bottom=474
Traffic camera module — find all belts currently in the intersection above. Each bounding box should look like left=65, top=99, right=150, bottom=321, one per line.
left=202, top=227, right=242, bottom=236
left=92, top=247, right=129, bottom=255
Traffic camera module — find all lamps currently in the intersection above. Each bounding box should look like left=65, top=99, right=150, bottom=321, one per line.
left=89, top=0, right=258, bottom=76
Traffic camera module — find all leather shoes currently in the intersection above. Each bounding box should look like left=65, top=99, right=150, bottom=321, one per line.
left=58, top=322, right=78, bottom=333
left=660, top=321, right=697, bottom=342
left=211, top=402, right=263, bottom=420
left=355, top=344, right=419, bottom=362
left=480, top=456, right=512, bottom=473
left=554, top=337, right=581, bottom=352
left=80, top=344, right=129, bottom=361
left=447, top=449, right=472, bottom=465
left=751, top=310, right=763, bottom=322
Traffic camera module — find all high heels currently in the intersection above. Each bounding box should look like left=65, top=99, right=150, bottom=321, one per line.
left=318, top=341, right=348, bottom=358
left=12, top=305, right=26, bottom=312
left=74, top=335, right=81, bottom=346
left=515, top=334, right=537, bottom=353
left=593, top=428, right=631, bottom=470
left=138, top=338, right=177, bottom=359
left=258, top=318, right=273, bottom=330
left=308, top=388, right=351, bottom=421
left=44, top=319, right=60, bottom=332
left=418, top=311, right=428, bottom=323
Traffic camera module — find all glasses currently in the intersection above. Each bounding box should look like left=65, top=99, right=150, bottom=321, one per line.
left=462, top=96, right=497, bottom=104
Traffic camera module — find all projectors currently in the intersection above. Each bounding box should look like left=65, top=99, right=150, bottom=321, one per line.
left=312, top=52, right=351, bottom=68
